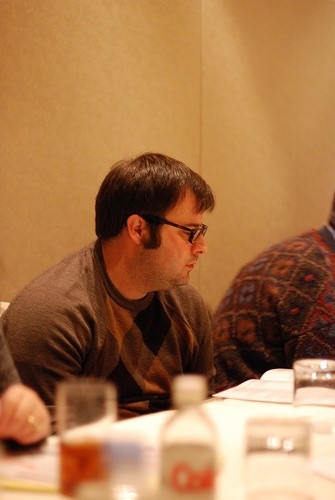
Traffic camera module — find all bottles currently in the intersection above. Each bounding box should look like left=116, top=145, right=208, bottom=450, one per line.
left=158, top=374, right=219, bottom=500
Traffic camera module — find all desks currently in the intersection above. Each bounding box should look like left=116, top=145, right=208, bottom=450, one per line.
left=0, top=397, right=334, bottom=499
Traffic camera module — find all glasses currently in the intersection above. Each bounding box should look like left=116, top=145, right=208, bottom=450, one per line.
left=141, top=213, right=208, bottom=243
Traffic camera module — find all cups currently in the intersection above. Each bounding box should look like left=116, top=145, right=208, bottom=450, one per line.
left=247, top=413, right=312, bottom=457
left=56, top=382, right=119, bottom=500
left=294, top=359, right=335, bottom=405
